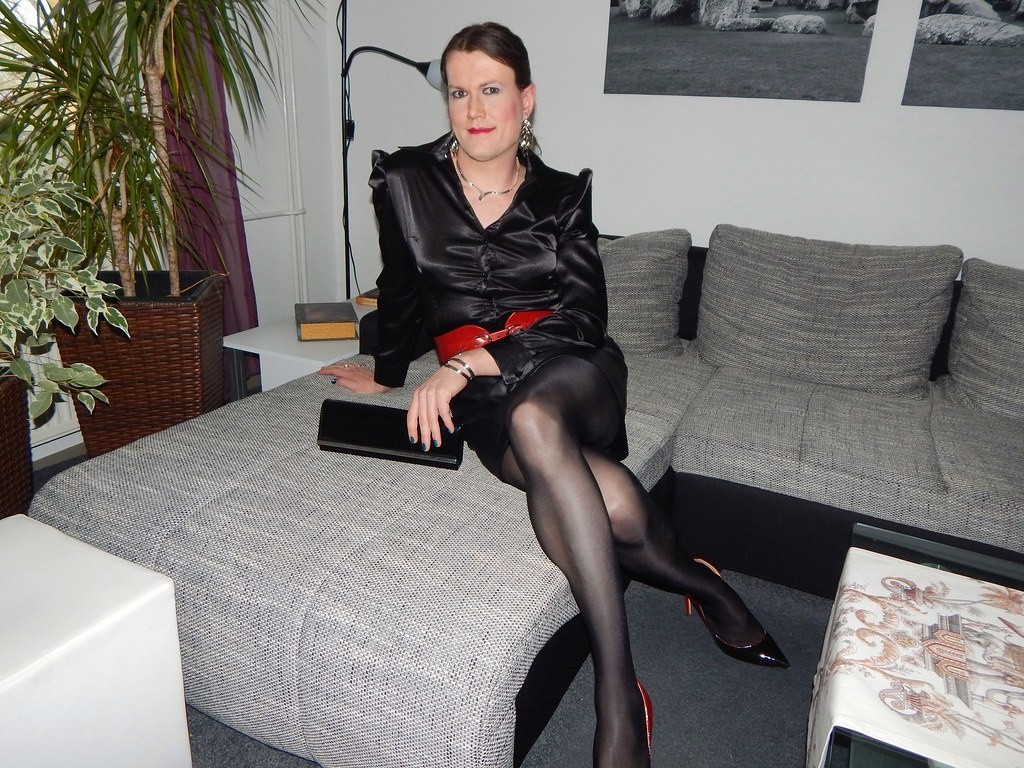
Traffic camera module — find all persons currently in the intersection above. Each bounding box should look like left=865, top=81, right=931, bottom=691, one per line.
left=317, top=22, right=789, bottom=768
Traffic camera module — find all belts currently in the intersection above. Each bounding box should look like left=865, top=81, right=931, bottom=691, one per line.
left=434, top=311, right=554, bottom=365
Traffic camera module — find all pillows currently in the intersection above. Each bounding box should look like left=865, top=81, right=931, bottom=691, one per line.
left=947, top=256, right=1024, bottom=426
left=695, top=226, right=964, bottom=402
left=596, top=229, right=693, bottom=360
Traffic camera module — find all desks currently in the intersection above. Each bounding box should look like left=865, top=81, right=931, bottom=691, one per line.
left=804, top=521, right=1024, bottom=768
left=222, top=291, right=380, bottom=395
left=0, top=511, right=195, bottom=768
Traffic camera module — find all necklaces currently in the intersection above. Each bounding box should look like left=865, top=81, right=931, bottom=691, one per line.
left=456, top=156, right=520, bottom=201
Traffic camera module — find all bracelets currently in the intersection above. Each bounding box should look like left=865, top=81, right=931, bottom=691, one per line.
left=444, top=357, right=475, bottom=381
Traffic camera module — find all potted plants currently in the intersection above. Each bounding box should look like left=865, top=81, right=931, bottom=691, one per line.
left=0, top=0, right=322, bottom=520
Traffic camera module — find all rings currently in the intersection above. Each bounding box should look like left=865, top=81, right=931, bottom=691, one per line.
left=357, top=365, right=361, bottom=368
left=344, top=365, right=349, bottom=368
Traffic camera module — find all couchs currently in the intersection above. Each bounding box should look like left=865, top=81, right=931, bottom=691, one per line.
left=25, top=227, right=718, bottom=768
left=671, top=222, right=1024, bottom=604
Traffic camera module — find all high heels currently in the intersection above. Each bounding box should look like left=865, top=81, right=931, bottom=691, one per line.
left=634, top=678, right=653, bottom=768
left=686, top=558, right=791, bottom=671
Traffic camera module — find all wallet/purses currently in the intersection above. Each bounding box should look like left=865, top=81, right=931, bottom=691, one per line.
left=317, top=400, right=467, bottom=470
